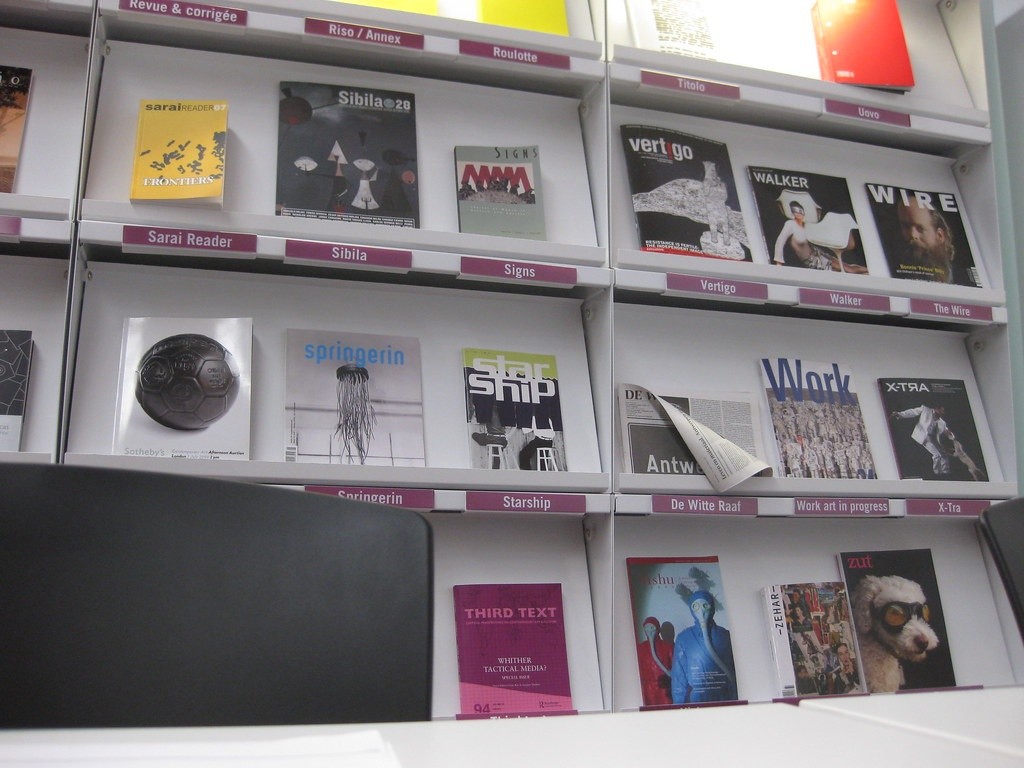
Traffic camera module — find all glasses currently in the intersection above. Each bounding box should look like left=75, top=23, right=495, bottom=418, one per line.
left=880, top=601, right=932, bottom=632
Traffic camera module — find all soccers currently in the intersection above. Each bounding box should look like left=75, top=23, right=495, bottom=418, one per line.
left=133, top=332, right=241, bottom=432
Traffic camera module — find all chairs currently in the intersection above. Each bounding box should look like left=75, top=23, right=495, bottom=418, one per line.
left=0, top=459, right=438, bottom=736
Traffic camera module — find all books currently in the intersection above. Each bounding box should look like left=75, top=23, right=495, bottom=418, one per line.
left=626, top=555, right=738, bottom=705
left=0, top=65, right=33, bottom=194
left=341, top=0, right=917, bottom=98
left=621, top=124, right=983, bottom=287
left=462, top=348, right=568, bottom=472
left=0, top=329, right=34, bottom=452
left=621, top=355, right=990, bottom=494
left=836, top=548, right=956, bottom=693
left=110, top=315, right=426, bottom=468
left=761, top=582, right=864, bottom=697
left=128, top=81, right=547, bottom=240
left=454, top=582, right=573, bottom=713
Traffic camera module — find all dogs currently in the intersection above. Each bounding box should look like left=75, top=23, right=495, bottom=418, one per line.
left=849, top=573, right=940, bottom=693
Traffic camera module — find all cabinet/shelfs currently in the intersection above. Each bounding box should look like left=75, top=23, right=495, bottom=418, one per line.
left=1, top=0, right=1024, bottom=720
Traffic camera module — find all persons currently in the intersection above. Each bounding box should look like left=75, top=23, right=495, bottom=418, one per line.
left=891, top=402, right=955, bottom=475
left=781, top=582, right=860, bottom=695
left=472, top=405, right=555, bottom=470
left=894, top=196, right=977, bottom=287
left=774, top=201, right=868, bottom=274
